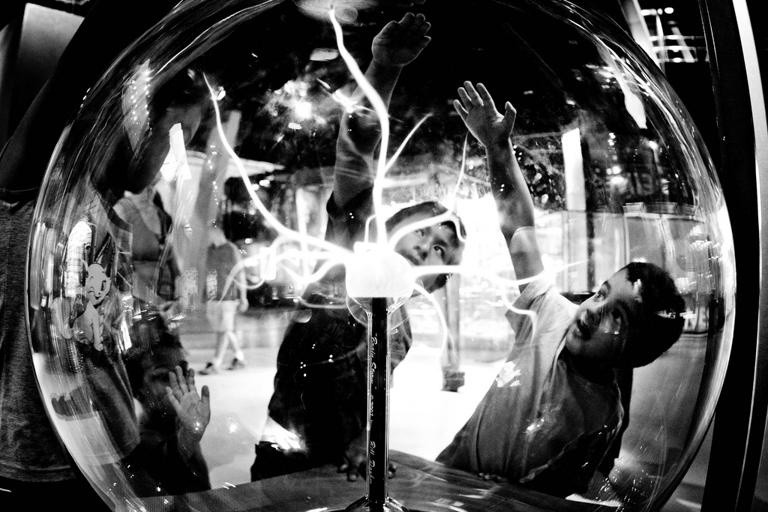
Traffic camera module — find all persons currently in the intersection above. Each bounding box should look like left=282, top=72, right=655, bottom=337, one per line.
left=250, top=11, right=469, bottom=482
left=434, top=80, right=687, bottom=500
left=198, top=220, right=248, bottom=376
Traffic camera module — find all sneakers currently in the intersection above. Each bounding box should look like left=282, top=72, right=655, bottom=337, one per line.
left=227, top=357, right=245, bottom=371
left=199, top=361, right=219, bottom=375
